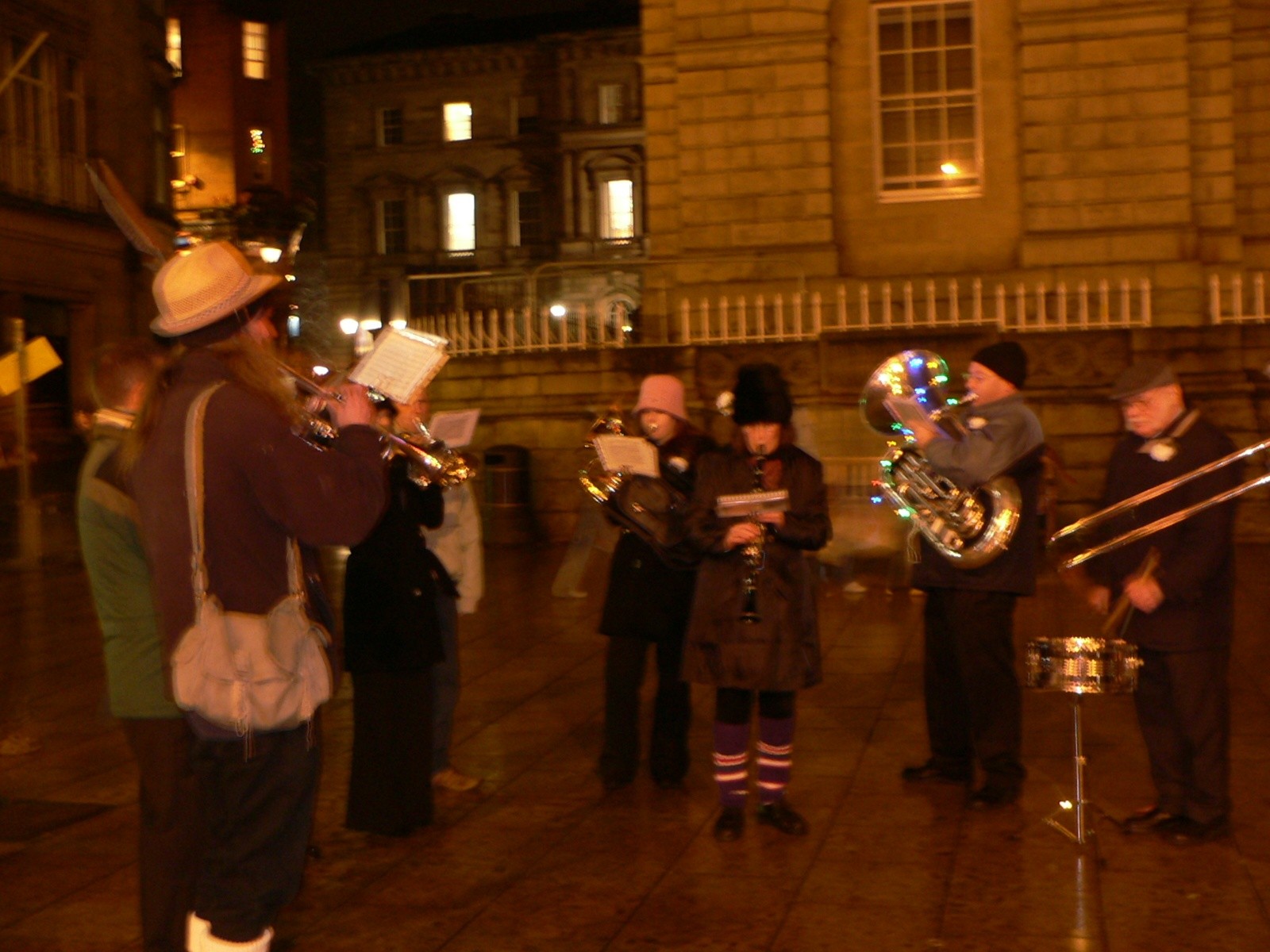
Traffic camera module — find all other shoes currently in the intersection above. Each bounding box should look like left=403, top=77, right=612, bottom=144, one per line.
left=758, top=803, right=810, bottom=838
left=717, top=806, right=744, bottom=842
left=432, top=768, right=481, bottom=791
left=650, top=770, right=686, bottom=790
left=900, top=760, right=975, bottom=784
left=1166, top=813, right=1229, bottom=848
left=603, top=767, right=632, bottom=790
left=344, top=810, right=414, bottom=838
left=400, top=800, right=433, bottom=825
left=972, top=785, right=1020, bottom=808
left=1122, top=807, right=1184, bottom=835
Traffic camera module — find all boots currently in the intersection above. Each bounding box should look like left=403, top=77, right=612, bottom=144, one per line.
left=185, top=912, right=273, bottom=952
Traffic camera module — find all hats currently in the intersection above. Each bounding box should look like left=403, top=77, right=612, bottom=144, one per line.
left=1108, top=361, right=1177, bottom=400
left=971, top=337, right=1028, bottom=391
left=631, top=374, right=690, bottom=424
left=150, top=239, right=282, bottom=338
left=733, top=360, right=792, bottom=429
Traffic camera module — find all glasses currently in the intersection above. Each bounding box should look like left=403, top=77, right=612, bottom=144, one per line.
left=961, top=372, right=1013, bottom=394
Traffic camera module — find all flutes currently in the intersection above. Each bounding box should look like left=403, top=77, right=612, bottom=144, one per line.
left=730, top=447, right=766, bottom=630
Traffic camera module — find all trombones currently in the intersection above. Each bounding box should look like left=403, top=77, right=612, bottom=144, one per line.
left=1047, top=430, right=1270, bottom=576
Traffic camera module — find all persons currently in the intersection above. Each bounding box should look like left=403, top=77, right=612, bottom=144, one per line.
left=72, top=212, right=477, bottom=952
left=579, top=372, right=712, bottom=794
left=896, top=342, right=1051, bottom=815
left=1081, top=358, right=1240, bottom=852
left=683, top=363, right=834, bottom=842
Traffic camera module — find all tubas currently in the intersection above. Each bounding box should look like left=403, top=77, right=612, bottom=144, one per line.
left=577, top=404, right=673, bottom=555
left=855, top=349, right=1020, bottom=571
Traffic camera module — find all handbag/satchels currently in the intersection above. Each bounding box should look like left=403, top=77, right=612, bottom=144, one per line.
left=170, top=591, right=331, bottom=735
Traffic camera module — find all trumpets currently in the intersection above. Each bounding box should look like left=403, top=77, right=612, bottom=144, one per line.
left=271, top=348, right=475, bottom=493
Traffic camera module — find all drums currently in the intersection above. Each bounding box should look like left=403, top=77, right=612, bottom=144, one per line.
left=1016, top=634, right=1144, bottom=698
